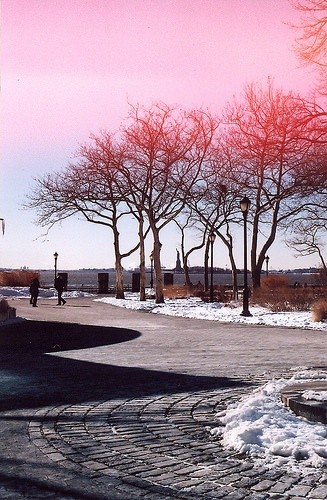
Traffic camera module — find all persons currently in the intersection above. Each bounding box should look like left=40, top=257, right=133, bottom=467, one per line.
left=55, top=274, right=66, bottom=305
left=30, top=273, right=39, bottom=307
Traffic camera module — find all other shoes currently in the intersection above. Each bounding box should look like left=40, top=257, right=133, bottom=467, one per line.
left=33, top=303, right=38, bottom=307
left=30, top=300, right=33, bottom=304
left=62, top=301, right=66, bottom=306
left=57, top=304, right=61, bottom=306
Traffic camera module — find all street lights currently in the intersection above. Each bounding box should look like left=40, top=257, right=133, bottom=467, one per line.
left=54, top=252, right=59, bottom=288
left=239, top=194, right=252, bottom=316
left=150, top=254, right=153, bottom=289
left=265, top=255, right=270, bottom=275
left=208, top=232, right=217, bottom=303
